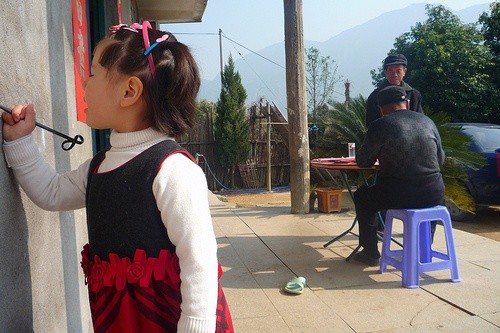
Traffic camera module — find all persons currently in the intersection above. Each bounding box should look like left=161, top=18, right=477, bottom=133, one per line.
left=0, top=20, right=234, bottom=333
left=353, top=83, right=446, bottom=267
left=365, top=54, right=424, bottom=133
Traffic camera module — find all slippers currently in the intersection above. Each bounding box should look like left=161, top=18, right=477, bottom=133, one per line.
left=284, top=277, right=306, bottom=294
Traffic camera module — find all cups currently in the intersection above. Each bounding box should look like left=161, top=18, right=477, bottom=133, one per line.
left=347, top=142, right=356, bottom=158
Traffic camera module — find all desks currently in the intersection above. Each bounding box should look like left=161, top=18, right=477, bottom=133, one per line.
left=311, top=157, right=404, bottom=262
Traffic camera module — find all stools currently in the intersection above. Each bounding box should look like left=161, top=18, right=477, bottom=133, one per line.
left=379, top=205, right=462, bottom=288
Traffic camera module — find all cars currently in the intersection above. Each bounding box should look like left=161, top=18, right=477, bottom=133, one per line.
left=440, top=123, right=500, bottom=222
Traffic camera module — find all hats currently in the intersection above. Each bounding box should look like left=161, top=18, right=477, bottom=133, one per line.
left=384, top=54, right=408, bottom=68
left=377, top=86, right=407, bottom=106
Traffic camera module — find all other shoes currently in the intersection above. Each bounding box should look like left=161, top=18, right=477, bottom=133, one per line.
left=376, top=225, right=384, bottom=232
left=355, top=249, right=380, bottom=266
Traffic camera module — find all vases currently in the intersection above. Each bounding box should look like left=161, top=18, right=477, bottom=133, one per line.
left=313, top=186, right=344, bottom=214
